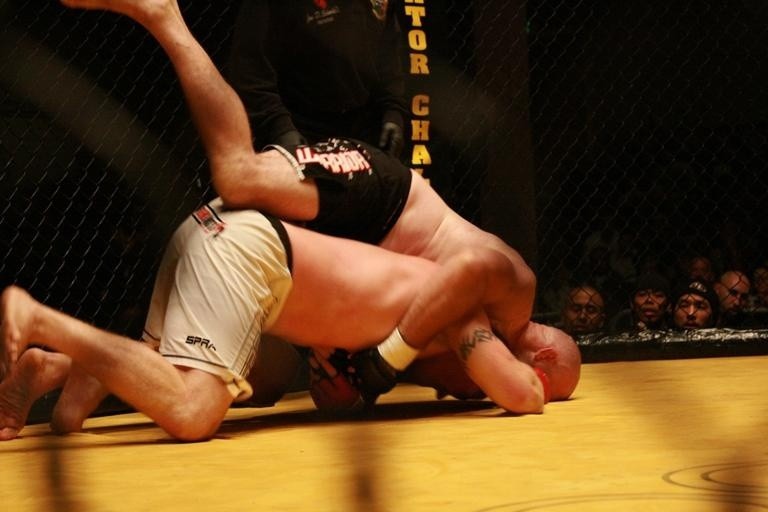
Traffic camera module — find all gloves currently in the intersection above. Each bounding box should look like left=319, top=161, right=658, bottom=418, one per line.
left=343, top=348, right=402, bottom=405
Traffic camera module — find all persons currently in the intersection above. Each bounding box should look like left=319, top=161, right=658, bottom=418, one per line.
left=49, top=1, right=583, bottom=437
left=0, top=192, right=548, bottom=443
left=523, top=238, right=768, bottom=343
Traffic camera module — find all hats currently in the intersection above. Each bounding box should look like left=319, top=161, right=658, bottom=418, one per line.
left=632, top=261, right=673, bottom=300
left=675, top=278, right=720, bottom=318
